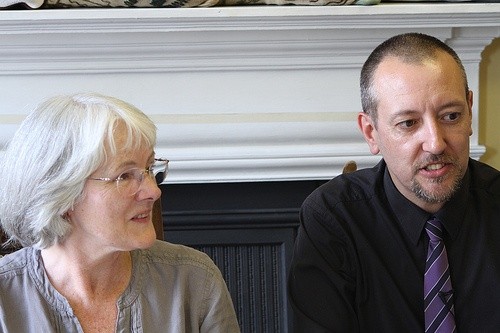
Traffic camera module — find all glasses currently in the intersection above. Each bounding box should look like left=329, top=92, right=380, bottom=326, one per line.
left=85, top=158, right=169, bottom=196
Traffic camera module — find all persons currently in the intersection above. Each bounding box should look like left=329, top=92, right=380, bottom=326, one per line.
left=292, top=31, right=500, bottom=333
left=1, top=92, right=240, bottom=333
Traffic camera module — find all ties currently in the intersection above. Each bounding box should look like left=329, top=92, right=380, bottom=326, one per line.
left=421, top=215, right=459, bottom=333
